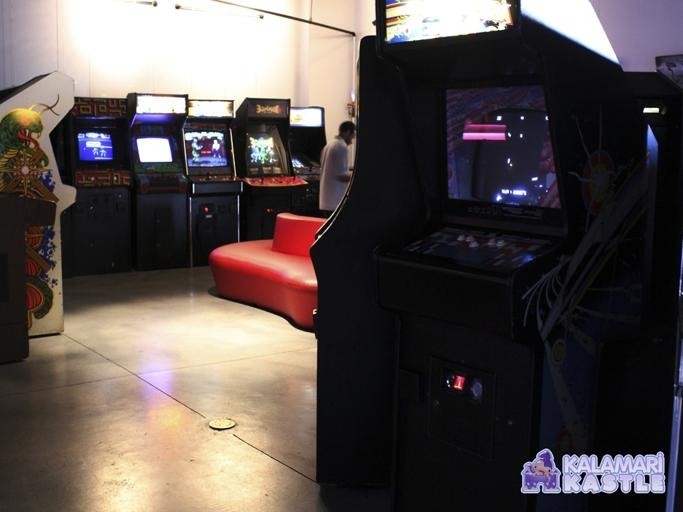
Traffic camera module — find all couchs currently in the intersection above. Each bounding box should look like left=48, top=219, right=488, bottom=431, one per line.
left=208, top=213, right=328, bottom=331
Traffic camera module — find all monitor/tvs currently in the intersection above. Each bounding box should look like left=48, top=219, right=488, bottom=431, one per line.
left=242, top=131, right=279, bottom=174
left=435, top=77, right=568, bottom=236
left=134, top=135, right=176, bottom=165
left=184, top=129, right=231, bottom=169
left=71, top=126, right=121, bottom=167
left=288, top=136, right=323, bottom=170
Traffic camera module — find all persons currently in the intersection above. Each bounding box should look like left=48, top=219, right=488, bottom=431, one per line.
left=211, top=139, right=221, bottom=158
left=190, top=137, right=203, bottom=163
left=318, top=120, right=355, bottom=216
